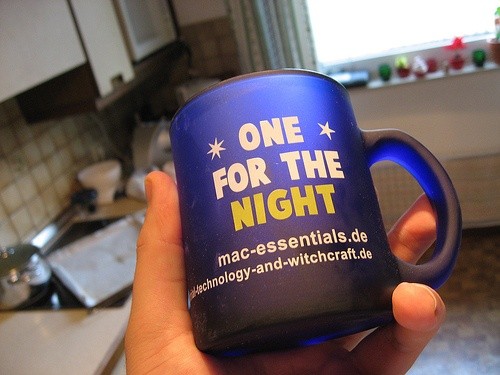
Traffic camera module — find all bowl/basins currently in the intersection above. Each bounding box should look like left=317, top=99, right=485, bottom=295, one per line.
left=77, top=159, right=122, bottom=192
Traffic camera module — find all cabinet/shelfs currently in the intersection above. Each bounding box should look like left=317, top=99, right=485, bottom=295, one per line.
left=0, top=0, right=180, bottom=124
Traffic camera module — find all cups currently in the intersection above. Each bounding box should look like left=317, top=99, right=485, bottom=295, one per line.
left=169, top=69, right=462, bottom=356
